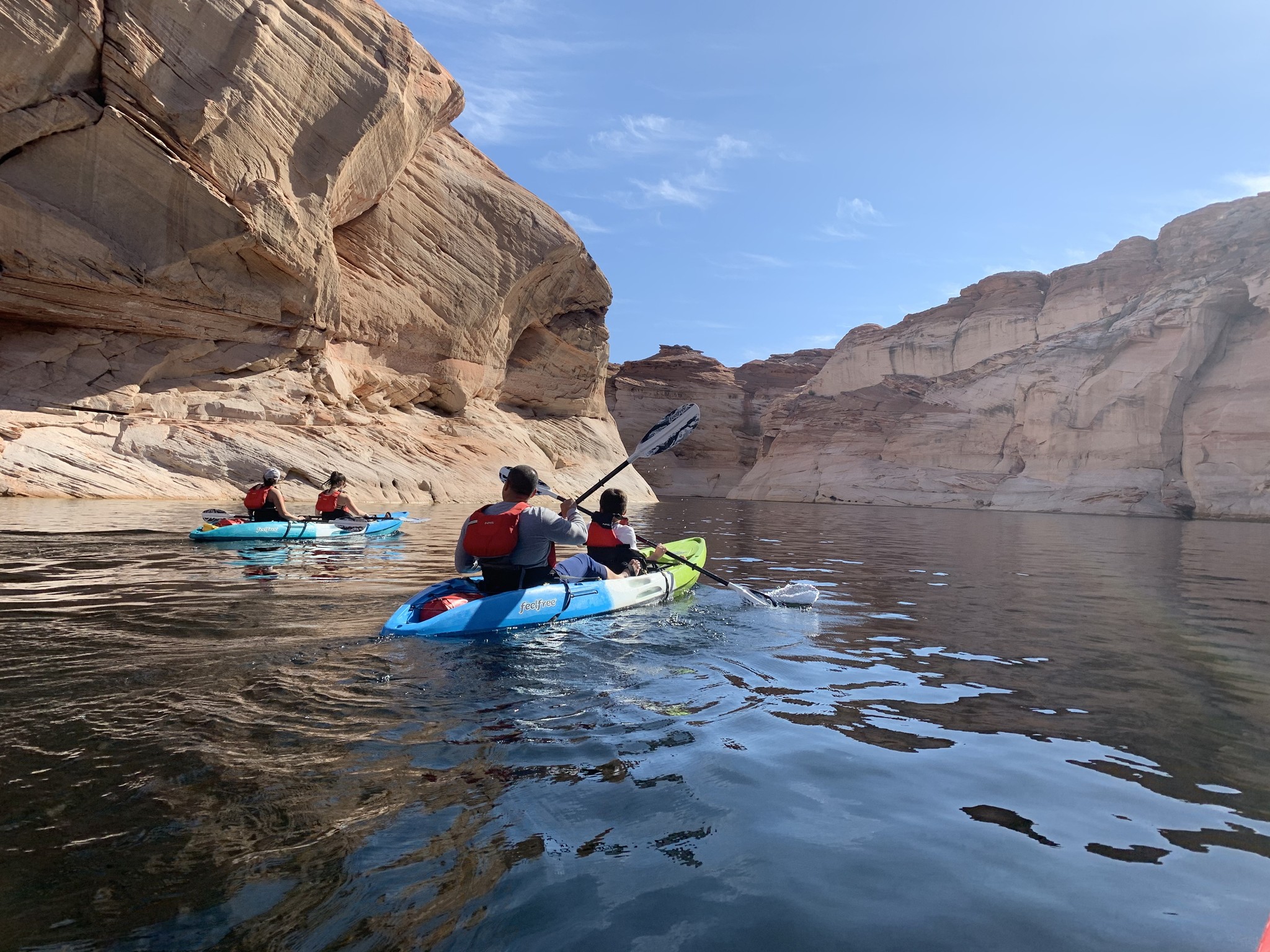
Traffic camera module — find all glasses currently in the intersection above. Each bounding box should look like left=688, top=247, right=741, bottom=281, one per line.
left=343, top=481, right=347, bottom=488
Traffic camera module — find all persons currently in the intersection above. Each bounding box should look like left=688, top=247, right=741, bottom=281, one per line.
left=247, top=467, right=312, bottom=523
left=586, top=487, right=686, bottom=574
left=314, top=471, right=392, bottom=522
left=455, top=464, right=642, bottom=595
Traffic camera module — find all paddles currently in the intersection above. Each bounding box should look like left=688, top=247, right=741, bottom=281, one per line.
left=202, top=508, right=367, bottom=532
left=294, top=515, right=432, bottom=523
left=498, top=466, right=776, bottom=608
left=557, top=402, right=701, bottom=517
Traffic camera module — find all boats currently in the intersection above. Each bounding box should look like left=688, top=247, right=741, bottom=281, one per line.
left=378, top=534, right=708, bottom=641
left=188, top=510, right=409, bottom=541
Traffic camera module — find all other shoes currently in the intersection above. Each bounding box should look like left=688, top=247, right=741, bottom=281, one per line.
left=382, top=512, right=391, bottom=521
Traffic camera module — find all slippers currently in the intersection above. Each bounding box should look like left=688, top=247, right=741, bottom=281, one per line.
left=625, top=560, right=643, bottom=577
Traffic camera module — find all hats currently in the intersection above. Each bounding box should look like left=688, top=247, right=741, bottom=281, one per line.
left=264, top=467, right=280, bottom=484
